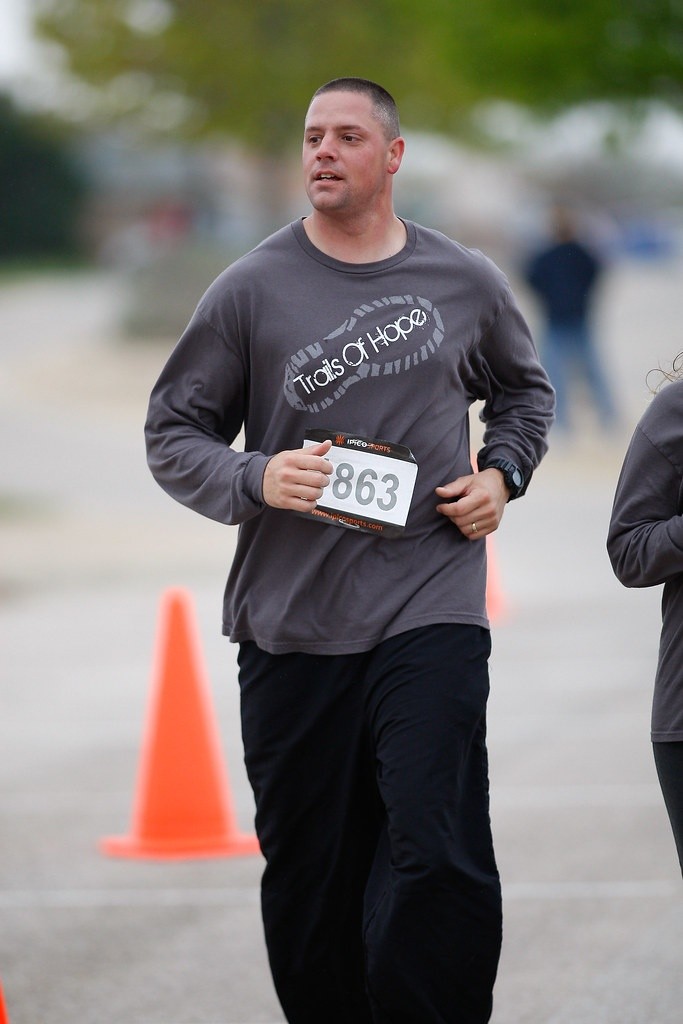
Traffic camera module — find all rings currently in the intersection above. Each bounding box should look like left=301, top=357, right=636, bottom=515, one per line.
left=471, top=522, right=478, bottom=533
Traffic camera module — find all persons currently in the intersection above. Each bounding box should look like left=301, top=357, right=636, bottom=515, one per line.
left=144, top=78, right=557, bottom=1023
left=608, top=346, right=683, bottom=880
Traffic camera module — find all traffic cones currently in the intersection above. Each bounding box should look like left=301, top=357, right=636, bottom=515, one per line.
left=91, top=587, right=265, bottom=861
